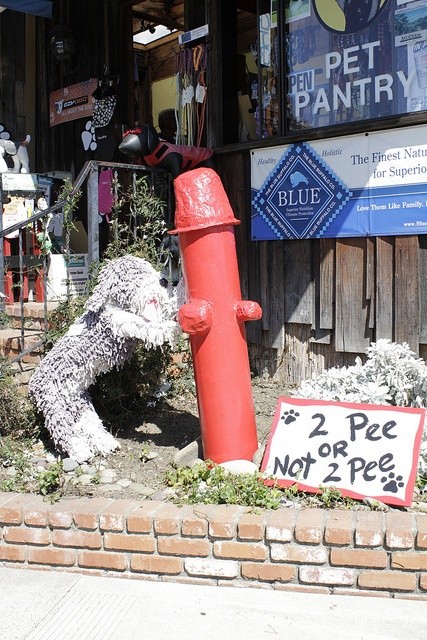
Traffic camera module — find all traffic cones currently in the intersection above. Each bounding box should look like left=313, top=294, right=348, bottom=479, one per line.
left=166, top=166, right=263, bottom=471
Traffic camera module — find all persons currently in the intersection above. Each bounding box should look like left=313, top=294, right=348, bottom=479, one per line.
left=156, top=109, right=189, bottom=145
